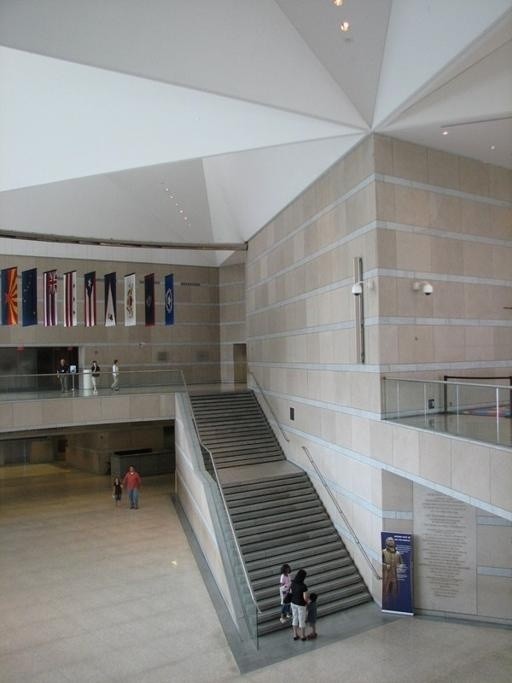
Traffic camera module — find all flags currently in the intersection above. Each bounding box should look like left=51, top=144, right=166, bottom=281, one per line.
left=1, top=266, right=176, bottom=327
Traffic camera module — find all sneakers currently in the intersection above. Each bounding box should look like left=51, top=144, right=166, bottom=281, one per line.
left=312, top=633, right=317, bottom=639
left=307, top=634, right=311, bottom=640
left=135, top=507, right=138, bottom=509
left=110, top=388, right=113, bottom=391
left=130, top=506, right=134, bottom=509
left=293, top=635, right=300, bottom=641
left=280, top=617, right=286, bottom=625
left=115, top=389, right=119, bottom=391
left=286, top=615, right=292, bottom=620
left=301, top=636, right=307, bottom=642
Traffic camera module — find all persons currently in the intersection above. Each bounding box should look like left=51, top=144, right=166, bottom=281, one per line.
left=306, top=593, right=318, bottom=639
left=110, top=359, right=120, bottom=392
left=279, top=563, right=292, bottom=624
left=90, top=360, right=100, bottom=396
left=121, top=464, right=144, bottom=509
left=57, top=359, right=71, bottom=393
left=112, top=476, right=122, bottom=507
left=382, top=537, right=404, bottom=611
left=289, top=569, right=309, bottom=641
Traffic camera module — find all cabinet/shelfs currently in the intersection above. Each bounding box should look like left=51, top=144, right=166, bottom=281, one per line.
left=109, top=450, right=176, bottom=479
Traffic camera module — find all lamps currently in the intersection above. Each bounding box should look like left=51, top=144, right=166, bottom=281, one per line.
left=411, top=278, right=433, bottom=296
left=353, top=256, right=364, bottom=363
left=352, top=280, right=374, bottom=295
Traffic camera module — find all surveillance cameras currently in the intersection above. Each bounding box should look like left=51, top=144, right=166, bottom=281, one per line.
left=422, top=284, right=433, bottom=296
left=352, top=284, right=362, bottom=297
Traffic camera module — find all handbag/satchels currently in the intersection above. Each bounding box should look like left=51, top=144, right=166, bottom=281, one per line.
left=283, top=586, right=293, bottom=604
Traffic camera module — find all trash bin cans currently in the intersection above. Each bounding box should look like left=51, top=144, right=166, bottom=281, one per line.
left=83, top=369, right=93, bottom=390
